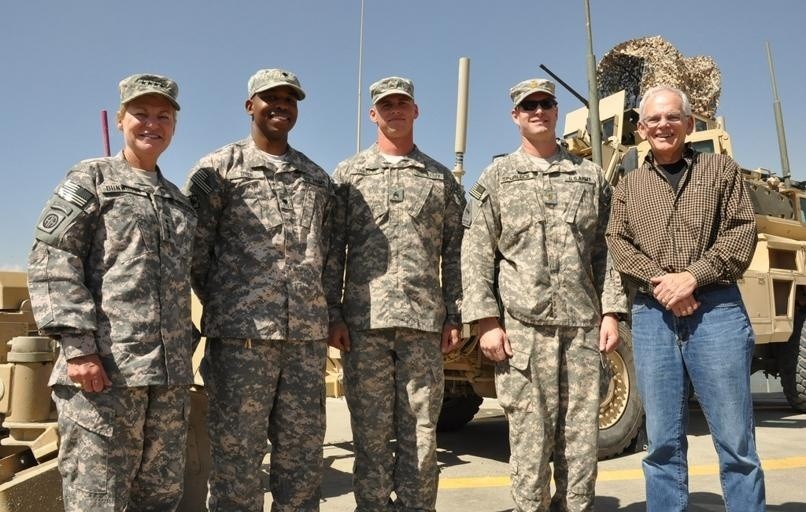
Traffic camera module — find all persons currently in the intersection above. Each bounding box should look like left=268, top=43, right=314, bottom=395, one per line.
left=24, top=70, right=202, bottom=512
left=604, top=82, right=772, bottom=511
left=458, top=76, right=631, bottom=511
left=326, top=75, right=468, bottom=512
left=180, top=66, right=339, bottom=512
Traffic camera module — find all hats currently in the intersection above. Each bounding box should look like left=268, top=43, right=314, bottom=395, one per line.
left=247, top=68, right=305, bottom=100
left=118, top=73, right=180, bottom=110
left=368, top=76, right=414, bottom=105
left=509, top=78, right=555, bottom=109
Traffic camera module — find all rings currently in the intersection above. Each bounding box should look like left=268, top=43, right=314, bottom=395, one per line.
left=74, top=382, right=83, bottom=388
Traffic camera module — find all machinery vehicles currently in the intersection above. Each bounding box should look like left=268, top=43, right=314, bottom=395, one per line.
left=326, top=2, right=806, bottom=461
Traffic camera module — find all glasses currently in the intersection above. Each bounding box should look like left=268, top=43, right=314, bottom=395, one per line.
left=519, top=97, right=557, bottom=111
left=641, top=112, right=685, bottom=129
